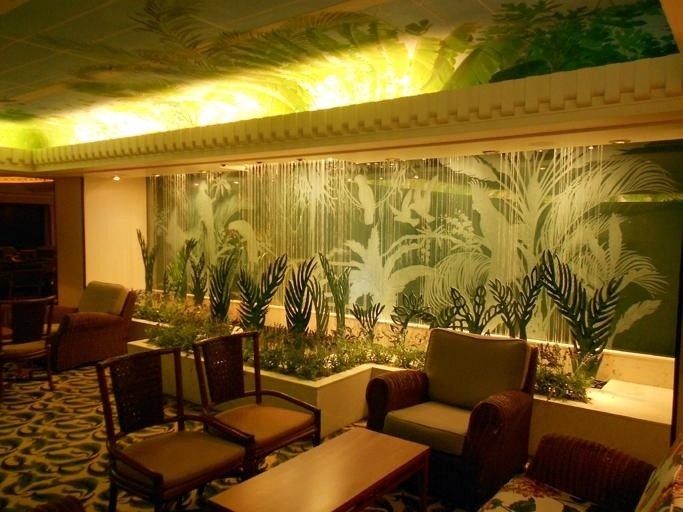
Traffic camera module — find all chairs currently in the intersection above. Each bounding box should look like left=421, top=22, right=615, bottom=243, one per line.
left=365, top=346, right=538, bottom=511
left=32, top=281, right=138, bottom=374
left=0, top=294, right=59, bottom=402
left=192, top=331, right=321, bottom=496
left=96, top=348, right=253, bottom=511
left=477, top=431, right=683, bottom=512
